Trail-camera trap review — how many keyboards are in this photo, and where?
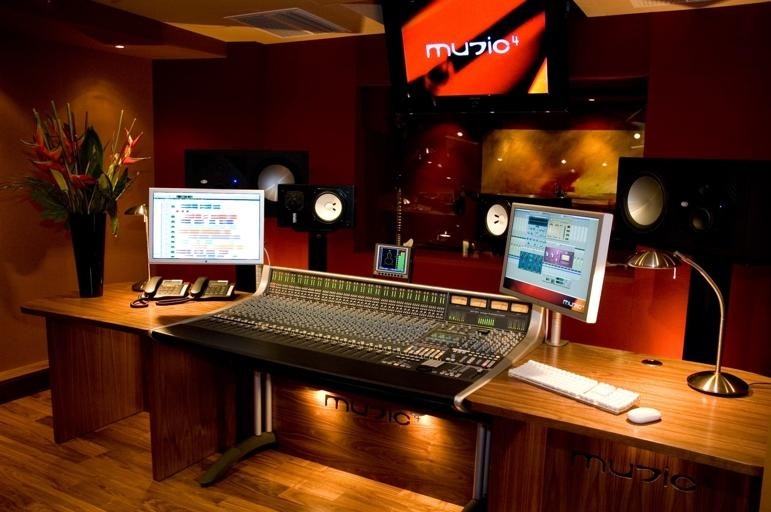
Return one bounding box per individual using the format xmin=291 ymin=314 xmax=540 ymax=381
xmin=508 ymin=360 xmax=641 ymax=415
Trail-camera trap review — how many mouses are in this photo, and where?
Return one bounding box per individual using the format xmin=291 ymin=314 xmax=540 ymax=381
xmin=628 ymin=407 xmax=660 ymax=424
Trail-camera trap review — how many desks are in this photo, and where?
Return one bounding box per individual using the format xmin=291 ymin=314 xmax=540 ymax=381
xmin=18 ymin=279 xmax=769 ymax=511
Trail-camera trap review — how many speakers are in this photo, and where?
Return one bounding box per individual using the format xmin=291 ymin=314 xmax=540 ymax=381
xmin=277 ymin=184 xmax=355 ymax=230
xmin=614 ymin=157 xmax=771 ymax=265
xmin=184 ymin=148 xmax=308 ymax=216
xmin=474 ymin=192 xmax=572 ymax=255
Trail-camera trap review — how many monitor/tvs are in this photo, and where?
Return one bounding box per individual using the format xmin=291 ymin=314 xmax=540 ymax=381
xmin=373 ymin=243 xmax=411 ymax=279
xmin=381 ymin=0 xmax=570 ymax=117
xmin=500 ymin=202 xmax=613 ymax=344
xmin=149 ymin=187 xmax=264 ymax=265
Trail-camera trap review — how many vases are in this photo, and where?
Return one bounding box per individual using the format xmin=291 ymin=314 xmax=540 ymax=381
xmin=67 ymin=212 xmax=107 ymax=297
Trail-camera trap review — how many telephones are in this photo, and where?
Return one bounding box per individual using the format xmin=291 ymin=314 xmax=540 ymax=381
xmin=188 ymin=276 xmax=237 ymax=300
xmin=140 ymin=275 xmax=190 ymax=299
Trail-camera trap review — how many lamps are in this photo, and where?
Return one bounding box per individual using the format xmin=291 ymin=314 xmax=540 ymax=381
xmin=627 ymin=247 xmax=749 ymax=397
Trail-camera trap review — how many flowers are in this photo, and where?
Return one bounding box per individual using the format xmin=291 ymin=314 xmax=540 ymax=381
xmin=0 ymin=99 xmax=152 ymax=241
